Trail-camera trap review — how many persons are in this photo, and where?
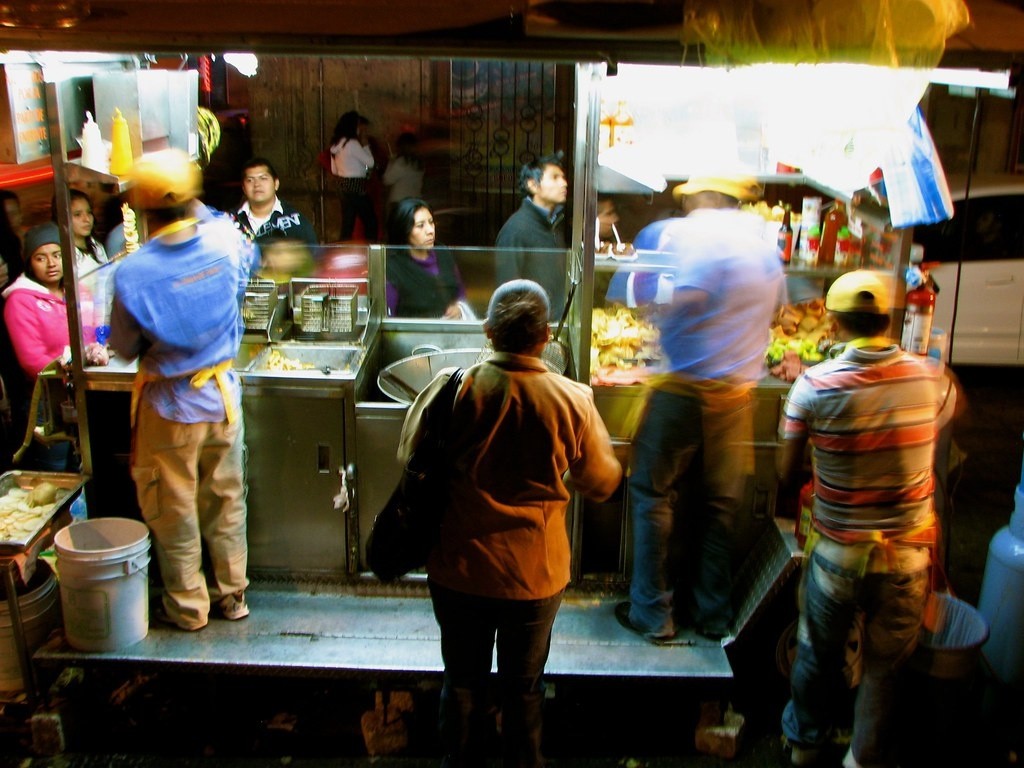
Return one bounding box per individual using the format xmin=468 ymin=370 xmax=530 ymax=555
xmin=109 ymin=148 xmax=260 ymax=634
xmin=396 ymin=279 xmax=622 ymax=768
xmin=0 ymin=187 xmax=221 ymax=471
xmin=603 ymin=171 xmax=787 ymax=642
xmin=778 ymin=271 xmax=956 ymax=768
xmin=231 ymin=155 xmax=328 ymax=295
xmin=383 ymin=197 xmax=465 ymax=320
xmin=495 ymin=151 xmax=619 ymax=322
xmin=329 ymin=110 xmax=424 ymax=247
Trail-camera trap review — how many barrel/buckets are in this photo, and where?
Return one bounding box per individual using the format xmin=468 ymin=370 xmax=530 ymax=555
xmin=0 ymin=559 xmax=57 ymax=705
xmin=54 ymin=518 xmax=151 ymax=651
xmin=795 ymin=480 xmax=815 ymax=549
xmin=903 ymin=593 xmax=989 ymax=756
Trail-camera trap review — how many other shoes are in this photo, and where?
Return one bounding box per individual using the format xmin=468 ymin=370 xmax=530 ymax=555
xmin=218 ymin=593 xmax=249 ymax=619
xmin=677 ymin=608 xmax=724 ymax=640
xmin=151 ymin=594 xmax=208 ymax=630
xmin=615 ymin=601 xmax=676 ymax=637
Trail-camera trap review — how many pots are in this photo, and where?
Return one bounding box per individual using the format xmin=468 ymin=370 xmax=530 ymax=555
xmin=377 ymin=344 xmax=561 ymax=406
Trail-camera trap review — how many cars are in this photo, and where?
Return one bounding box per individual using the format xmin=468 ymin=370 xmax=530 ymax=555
xmin=213 ymin=107 xmax=287 ymax=154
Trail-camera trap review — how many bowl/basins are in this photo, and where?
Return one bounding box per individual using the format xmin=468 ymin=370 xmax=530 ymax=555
xmin=769 ymin=223 xmax=801 ymax=255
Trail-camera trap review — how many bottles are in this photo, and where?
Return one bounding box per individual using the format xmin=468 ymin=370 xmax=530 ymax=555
xmin=81 ymin=112 xmax=109 ymax=176
xmin=834 ymin=226 xmax=851 ymax=265
xmin=804 ymin=226 xmax=819 ymax=266
xmin=776 ymin=205 xmax=793 ymax=263
xmin=818 ymin=200 xmax=848 ymax=264
xmin=909 ymin=245 xmax=924 ymax=270
xmin=929 ymin=328 xmax=948 ymax=370
xmin=850 ymin=220 xmax=898 ymax=269
xmin=111 ymin=108 xmax=134 ymax=175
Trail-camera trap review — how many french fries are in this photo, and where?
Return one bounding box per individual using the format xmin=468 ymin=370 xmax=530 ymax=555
xmin=269 ymin=350 xmax=315 ymax=371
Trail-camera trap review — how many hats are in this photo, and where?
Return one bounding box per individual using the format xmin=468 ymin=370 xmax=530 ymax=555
xmin=674 ymin=177 xmax=758 ymax=200
xmin=826 ymin=270 xmax=891 ymax=313
xmin=25 ymin=222 xmax=60 ymax=259
xmin=130 ymin=150 xmax=201 ymax=208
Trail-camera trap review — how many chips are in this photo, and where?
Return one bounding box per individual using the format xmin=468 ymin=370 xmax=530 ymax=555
xmin=0 ymin=488 xmax=56 ymax=542
xmin=771 ymin=297 xmax=831 ymax=344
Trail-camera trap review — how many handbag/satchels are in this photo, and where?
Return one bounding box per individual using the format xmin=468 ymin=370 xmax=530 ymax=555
xmin=366 ymin=368 xmax=465 ymax=585
xmin=441 ymin=301 xmax=477 ymax=322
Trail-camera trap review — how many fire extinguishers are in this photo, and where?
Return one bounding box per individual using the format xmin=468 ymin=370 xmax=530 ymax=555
xmin=902 ymin=263 xmax=941 ymax=358
